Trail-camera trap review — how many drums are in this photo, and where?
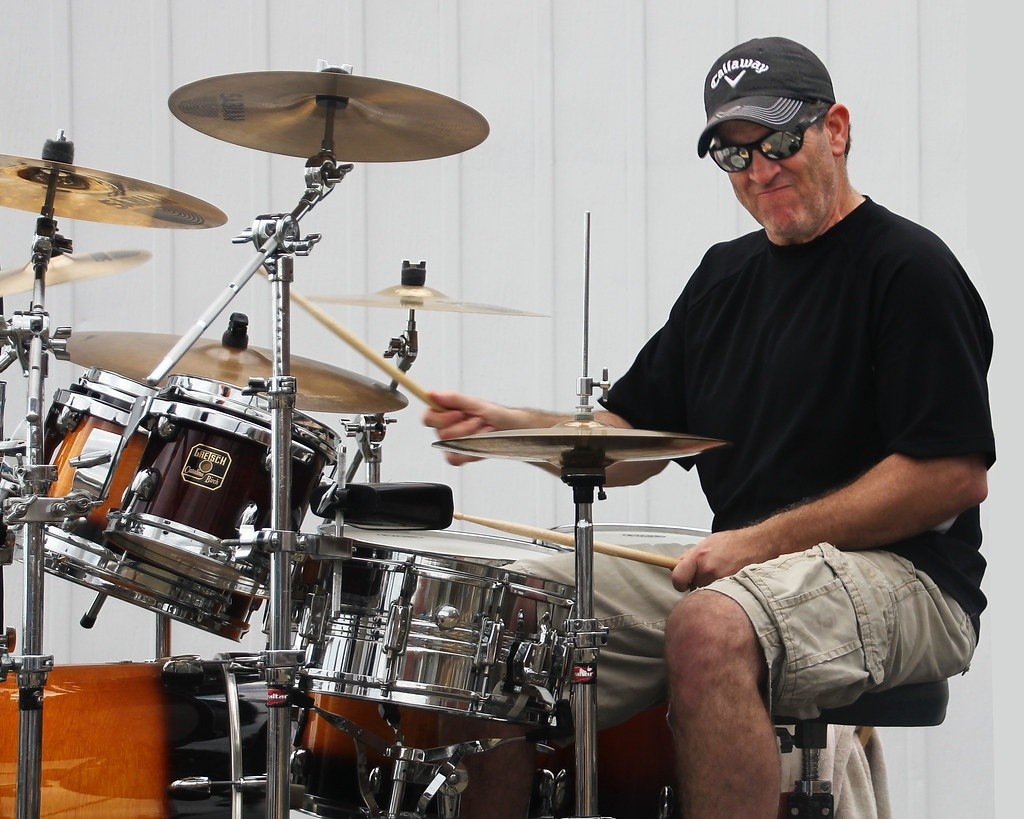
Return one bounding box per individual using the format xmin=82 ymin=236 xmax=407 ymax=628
xmin=528 ymin=523 xmax=720 ymax=818
xmin=274 ymin=532 xmax=579 ymax=731
xmin=287 ymin=691 xmax=556 ymax=819
xmin=108 ymin=371 xmax=347 ymax=601
xmin=9 ymin=366 xmax=260 ymax=645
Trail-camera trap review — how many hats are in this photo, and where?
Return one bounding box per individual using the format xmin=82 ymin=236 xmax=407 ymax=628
xmin=698 ymin=37 xmax=836 ymax=159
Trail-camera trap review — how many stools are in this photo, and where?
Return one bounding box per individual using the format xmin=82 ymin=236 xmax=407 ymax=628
xmin=771 ymin=678 xmax=949 ymax=819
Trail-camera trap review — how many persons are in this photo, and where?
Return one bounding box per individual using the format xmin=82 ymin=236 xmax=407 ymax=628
xmin=424 ymin=38 xmax=997 ymax=819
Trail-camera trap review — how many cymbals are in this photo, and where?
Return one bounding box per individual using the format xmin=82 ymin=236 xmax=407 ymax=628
xmin=433 ymin=416 xmax=729 ymax=461
xmin=288 ymin=283 xmax=551 ymax=318
xmin=0 ymin=250 xmax=153 ymax=293
xmin=55 ymin=329 xmax=407 ymax=416
xmin=0 ymin=153 xmax=230 ymax=230
xmin=166 ymin=69 xmax=491 ymax=163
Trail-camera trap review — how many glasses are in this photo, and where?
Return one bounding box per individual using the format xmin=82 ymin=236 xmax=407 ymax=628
xmin=709 ymin=107 xmax=828 ymax=173
